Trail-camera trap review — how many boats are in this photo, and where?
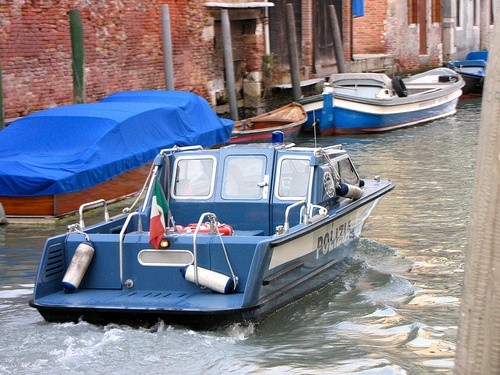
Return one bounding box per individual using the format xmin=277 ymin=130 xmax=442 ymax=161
xmin=29 ymin=131 xmax=397 ymax=326
xmin=0 ymin=90 xmax=307 ymax=224
xmin=446 ymin=51 xmax=487 ymax=95
xmin=297 ymin=67 xmax=466 ymax=134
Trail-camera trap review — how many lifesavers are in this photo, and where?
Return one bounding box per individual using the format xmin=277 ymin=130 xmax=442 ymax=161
xmin=170 ymin=223 xmax=235 ymax=236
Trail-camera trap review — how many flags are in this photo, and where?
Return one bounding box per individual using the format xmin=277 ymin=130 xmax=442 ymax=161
xmin=149 ymin=174 xmax=170 ymax=250
